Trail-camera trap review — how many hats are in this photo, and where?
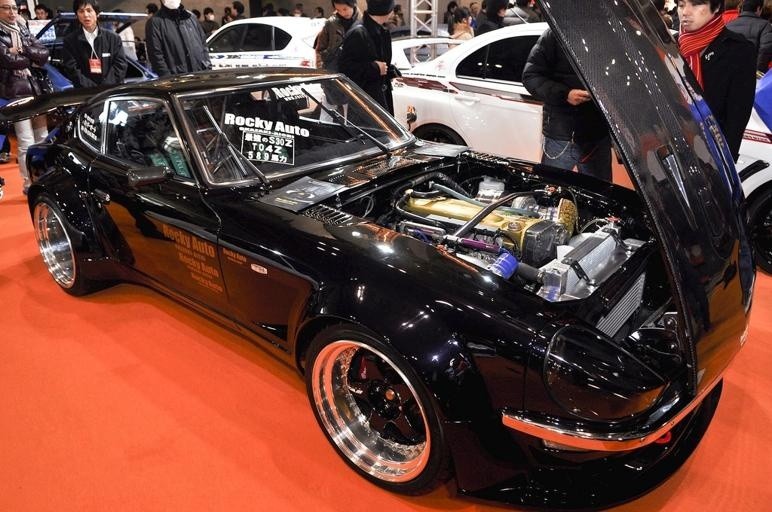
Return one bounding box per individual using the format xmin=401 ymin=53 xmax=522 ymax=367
xmin=365 ymin=1 xmax=395 ymax=16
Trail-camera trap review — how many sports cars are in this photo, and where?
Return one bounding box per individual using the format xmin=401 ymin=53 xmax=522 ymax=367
xmin=5 ymin=2 xmax=760 ymax=508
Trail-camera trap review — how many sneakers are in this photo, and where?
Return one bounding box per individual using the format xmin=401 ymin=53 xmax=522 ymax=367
xmin=1 ymin=151 xmax=11 ymax=163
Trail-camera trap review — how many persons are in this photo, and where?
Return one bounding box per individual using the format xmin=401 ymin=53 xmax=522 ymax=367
xmin=521 ymin=25 xmax=617 ymax=185
xmin=313 ymin=0 xmax=358 ymax=110
xmin=0 ymin=0 xmax=50 ymax=196
xmin=341 ymin=0 xmax=398 ymax=118
xmin=60 ymin=0 xmax=129 ymax=89
xmin=670 ymin=1 xmax=759 ymax=164
xmin=727 ymin=1 xmax=772 ymax=77
xmin=144 ymin=0 xmax=212 ymax=78
xmin=18 ymin=3 xmax=159 ymax=71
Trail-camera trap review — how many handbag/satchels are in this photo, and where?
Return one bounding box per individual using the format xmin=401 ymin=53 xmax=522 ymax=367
xmin=36 ymin=69 xmax=66 ymax=125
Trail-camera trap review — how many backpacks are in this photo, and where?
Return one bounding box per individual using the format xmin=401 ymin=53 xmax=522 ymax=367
xmin=320 ymin=41 xmax=350 ymax=106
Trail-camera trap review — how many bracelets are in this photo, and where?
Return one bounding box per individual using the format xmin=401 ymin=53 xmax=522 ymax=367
xmin=17 ymin=47 xmax=22 ymax=54
